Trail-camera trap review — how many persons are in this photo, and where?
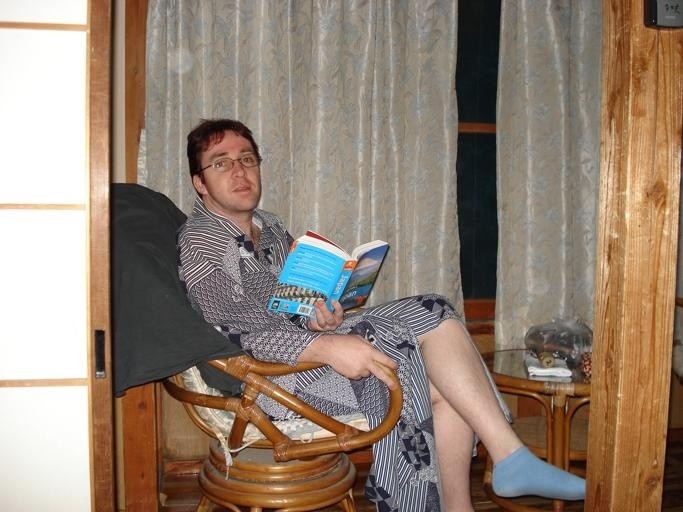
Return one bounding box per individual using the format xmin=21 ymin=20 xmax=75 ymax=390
xmin=174 ymin=118 xmax=590 ymax=511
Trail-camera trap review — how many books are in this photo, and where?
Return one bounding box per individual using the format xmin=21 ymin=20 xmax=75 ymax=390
xmin=265 ymin=228 xmax=389 ymax=321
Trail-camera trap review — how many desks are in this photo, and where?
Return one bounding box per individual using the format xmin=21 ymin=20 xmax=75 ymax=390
xmin=482 ymin=345 xmax=591 ymax=510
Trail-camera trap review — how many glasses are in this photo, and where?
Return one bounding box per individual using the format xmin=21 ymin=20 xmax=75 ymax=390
xmin=198 ymin=152 xmax=260 ymax=172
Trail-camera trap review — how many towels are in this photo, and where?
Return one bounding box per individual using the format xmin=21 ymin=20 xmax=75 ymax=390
xmin=525 ymin=356 xmax=572 ymax=379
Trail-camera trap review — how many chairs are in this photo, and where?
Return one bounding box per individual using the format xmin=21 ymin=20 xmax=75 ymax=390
xmin=109 ymin=182 xmax=402 ymax=511
